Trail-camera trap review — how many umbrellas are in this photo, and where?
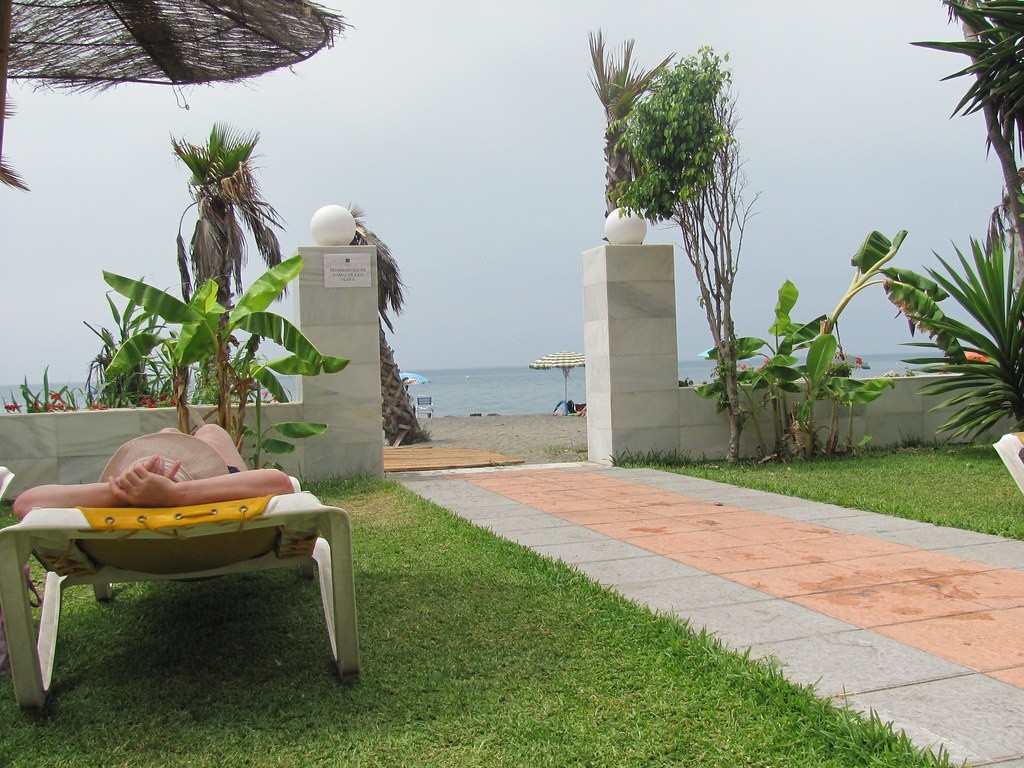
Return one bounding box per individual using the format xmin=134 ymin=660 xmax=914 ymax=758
xmin=831 ymin=351 xmax=870 ymax=370
xmin=529 ymin=350 xmax=586 ymax=415
xmin=698 ymin=347 xmax=715 ymax=357
xmin=399 ymin=371 xmax=432 ymax=394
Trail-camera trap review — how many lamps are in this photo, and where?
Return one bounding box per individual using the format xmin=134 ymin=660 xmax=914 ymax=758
xmin=604 ymin=207 xmax=647 ymax=244
xmin=311 ymin=205 xmax=356 ymax=247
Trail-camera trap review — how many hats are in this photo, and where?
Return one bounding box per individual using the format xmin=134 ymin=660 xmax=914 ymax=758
xmin=99 ymin=432 xmax=230 ymax=484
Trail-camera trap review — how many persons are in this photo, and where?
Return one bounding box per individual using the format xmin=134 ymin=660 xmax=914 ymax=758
xmin=13 ymin=423 xmax=295 ymax=520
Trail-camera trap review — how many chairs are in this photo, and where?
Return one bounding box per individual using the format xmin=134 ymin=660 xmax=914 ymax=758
xmin=0 ymin=476 xmax=361 ymax=708
xmin=416 ymin=397 xmax=433 ymax=419
